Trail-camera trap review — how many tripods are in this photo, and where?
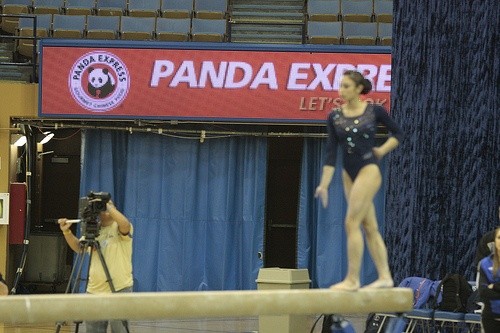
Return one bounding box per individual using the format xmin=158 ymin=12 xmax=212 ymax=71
xmin=54 ymin=218 xmax=132 ymax=333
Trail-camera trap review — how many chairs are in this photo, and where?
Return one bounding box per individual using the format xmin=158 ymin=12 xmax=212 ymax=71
xmin=302 ymin=0 xmax=393 ymax=47
xmin=0 ymin=0 xmax=230 ymax=65
xmin=374 ymin=276 xmax=485 ymax=333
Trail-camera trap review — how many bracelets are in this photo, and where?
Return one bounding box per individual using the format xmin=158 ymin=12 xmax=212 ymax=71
xmin=63 ymin=229 xmax=71 ymax=235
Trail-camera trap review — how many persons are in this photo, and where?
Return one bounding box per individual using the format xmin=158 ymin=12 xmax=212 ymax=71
xmin=315 ymin=70 xmax=404 ymax=292
xmin=476 ymin=227 xmax=500 ymax=333
xmin=58 ymin=200 xmax=134 ymax=333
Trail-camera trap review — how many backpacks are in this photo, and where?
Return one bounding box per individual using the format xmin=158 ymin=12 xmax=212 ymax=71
xmin=430 ymin=273 xmax=472 ymax=327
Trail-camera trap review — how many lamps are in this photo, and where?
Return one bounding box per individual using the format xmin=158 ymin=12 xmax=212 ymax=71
xmin=10 ymin=126 xmax=55 ymax=146
